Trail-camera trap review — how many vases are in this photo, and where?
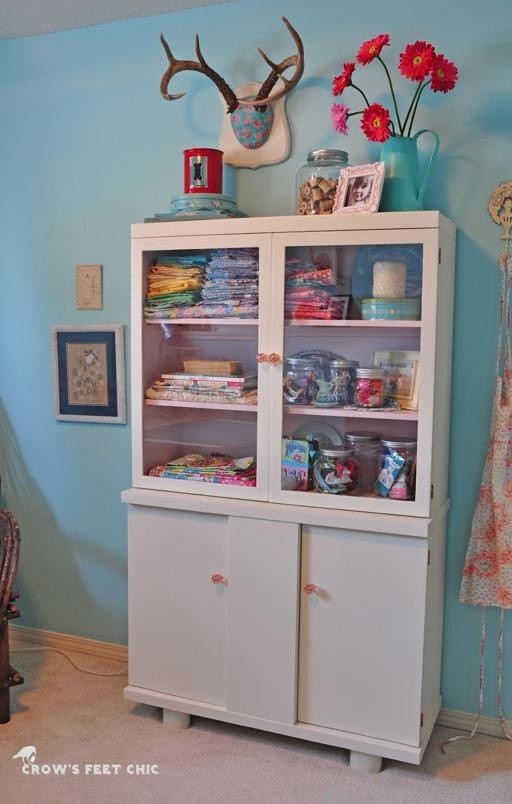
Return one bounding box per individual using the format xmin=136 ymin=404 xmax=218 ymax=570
xmin=363 ymin=132 xmax=445 ymax=210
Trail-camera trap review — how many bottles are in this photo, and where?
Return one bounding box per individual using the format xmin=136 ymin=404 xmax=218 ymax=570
xmin=345 ymin=431 xmax=380 ymax=491
xmin=379 ymin=438 xmax=416 ymax=500
xmin=328 ymin=360 xmax=358 ymax=404
xmin=354 ymin=368 xmax=385 ymax=408
xmin=283 ymin=356 xmax=324 ymax=405
xmin=312 ymin=447 xmax=359 ymax=494
xmin=294 ymin=150 xmax=357 ymax=214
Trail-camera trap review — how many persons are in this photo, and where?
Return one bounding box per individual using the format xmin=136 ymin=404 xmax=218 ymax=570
xmin=349 ymin=175 xmax=373 ymax=205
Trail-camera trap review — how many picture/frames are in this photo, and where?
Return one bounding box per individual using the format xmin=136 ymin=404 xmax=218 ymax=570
xmin=329 ymin=160 xmax=385 ymax=213
xmin=50 ymin=322 xmax=127 ymax=427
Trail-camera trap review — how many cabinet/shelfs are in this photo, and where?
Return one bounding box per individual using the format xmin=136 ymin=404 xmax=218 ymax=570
xmin=124 ymin=206 xmax=452 ymax=774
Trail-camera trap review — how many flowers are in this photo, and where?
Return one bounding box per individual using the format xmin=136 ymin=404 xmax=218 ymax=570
xmin=328 ymin=39 xmax=463 ymax=134
